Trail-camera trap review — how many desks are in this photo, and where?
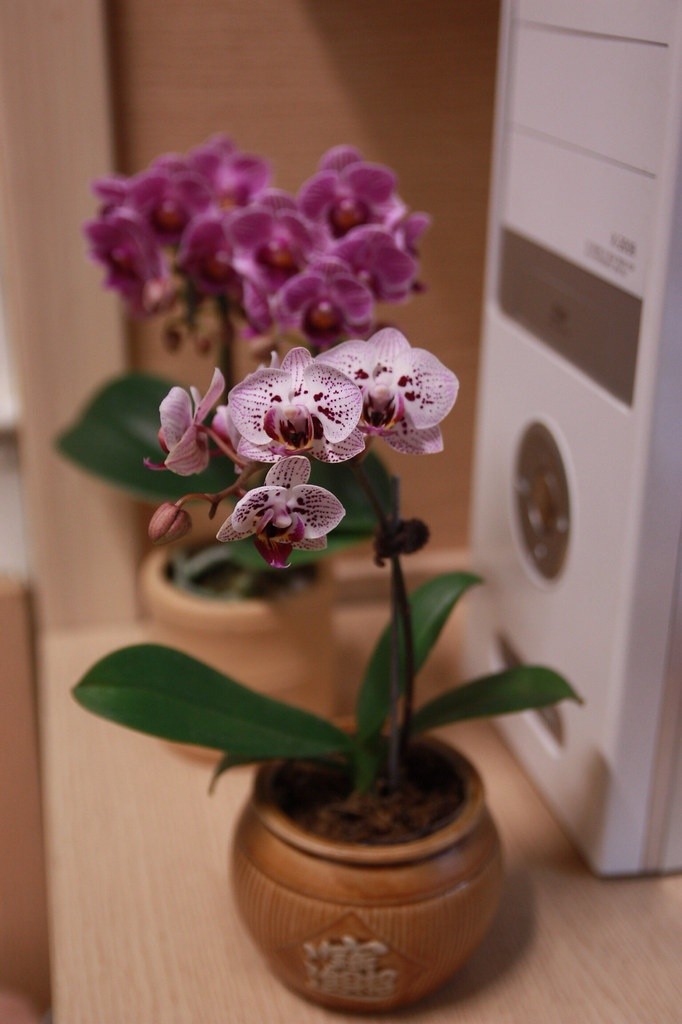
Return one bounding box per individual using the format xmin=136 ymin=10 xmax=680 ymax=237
xmin=36 ymin=553 xmax=682 ymax=1024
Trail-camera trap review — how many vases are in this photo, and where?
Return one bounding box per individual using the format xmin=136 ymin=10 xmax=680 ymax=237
xmin=137 ymin=526 xmax=344 ymax=775
xmin=226 ymin=716 xmax=508 ymax=1014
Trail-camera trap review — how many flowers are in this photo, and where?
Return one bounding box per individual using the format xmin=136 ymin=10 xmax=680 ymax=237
xmin=129 ymin=316 xmax=457 ymax=576
xmin=63 ymin=130 xmax=437 ymax=344
